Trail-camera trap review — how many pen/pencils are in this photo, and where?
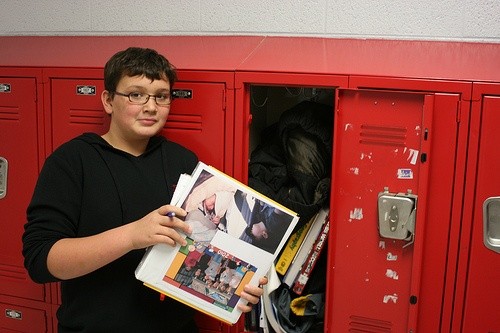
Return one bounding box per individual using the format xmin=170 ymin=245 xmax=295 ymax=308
xmin=167 ymin=212 xmax=176 ymax=217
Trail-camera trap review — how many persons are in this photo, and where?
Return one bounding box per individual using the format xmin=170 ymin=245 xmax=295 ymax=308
xmin=22 ymin=46 xmax=268 ymax=333
xmin=178 ymin=169 xmax=293 ymax=294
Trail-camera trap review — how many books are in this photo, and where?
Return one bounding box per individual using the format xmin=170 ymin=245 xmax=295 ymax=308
xmin=248 ymin=206 xmax=330 ymax=333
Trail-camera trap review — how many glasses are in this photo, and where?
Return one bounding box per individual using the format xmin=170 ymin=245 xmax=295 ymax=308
xmin=113 ymin=91 xmax=173 ymax=105
